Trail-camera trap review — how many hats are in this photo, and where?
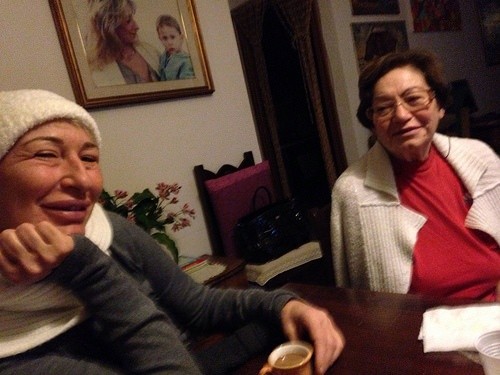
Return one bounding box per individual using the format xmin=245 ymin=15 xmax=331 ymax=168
xmin=0 ymin=89 xmax=103 ymax=159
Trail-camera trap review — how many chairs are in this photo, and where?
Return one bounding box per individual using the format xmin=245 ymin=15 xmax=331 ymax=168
xmin=193 ymin=152 xmax=328 ymax=292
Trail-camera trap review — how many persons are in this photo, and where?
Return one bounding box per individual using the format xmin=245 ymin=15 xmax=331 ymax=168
xmin=155 ymin=16 xmax=195 ymax=80
xmin=331 ymin=48 xmax=500 ymax=304
xmin=84 ymin=0 xmax=162 ymax=87
xmin=0 ymin=88 xmax=345 ymax=375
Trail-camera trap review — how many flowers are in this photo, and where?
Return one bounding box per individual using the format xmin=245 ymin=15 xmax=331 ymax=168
xmin=97 ymin=182 xmax=197 ymax=269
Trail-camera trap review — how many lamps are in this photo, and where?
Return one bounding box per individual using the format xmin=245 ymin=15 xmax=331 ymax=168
xmin=446 ymin=80 xmax=479 ymax=135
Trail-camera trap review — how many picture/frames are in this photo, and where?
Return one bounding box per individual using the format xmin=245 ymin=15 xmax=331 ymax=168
xmin=49 ymin=0 xmax=216 ymax=110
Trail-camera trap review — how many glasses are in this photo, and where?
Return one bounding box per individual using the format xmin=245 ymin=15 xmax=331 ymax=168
xmin=366 ymin=88 xmax=435 ymax=120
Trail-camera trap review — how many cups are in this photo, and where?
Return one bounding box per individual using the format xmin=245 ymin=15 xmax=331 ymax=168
xmin=475 ymin=329 xmax=500 ymax=375
xmin=259 ymin=341 xmax=314 ymax=375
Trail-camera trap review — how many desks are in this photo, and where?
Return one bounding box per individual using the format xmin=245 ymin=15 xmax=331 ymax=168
xmin=181 ymin=255 xmax=246 ymax=290
xmin=187 ymin=283 xmax=500 ymax=375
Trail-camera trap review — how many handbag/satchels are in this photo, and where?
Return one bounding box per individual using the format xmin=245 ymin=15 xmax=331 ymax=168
xmin=232 ymin=196 xmax=314 ymax=265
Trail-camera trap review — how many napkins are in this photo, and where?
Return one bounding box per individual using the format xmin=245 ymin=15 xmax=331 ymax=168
xmin=417 ymin=304 xmax=500 ymax=353
